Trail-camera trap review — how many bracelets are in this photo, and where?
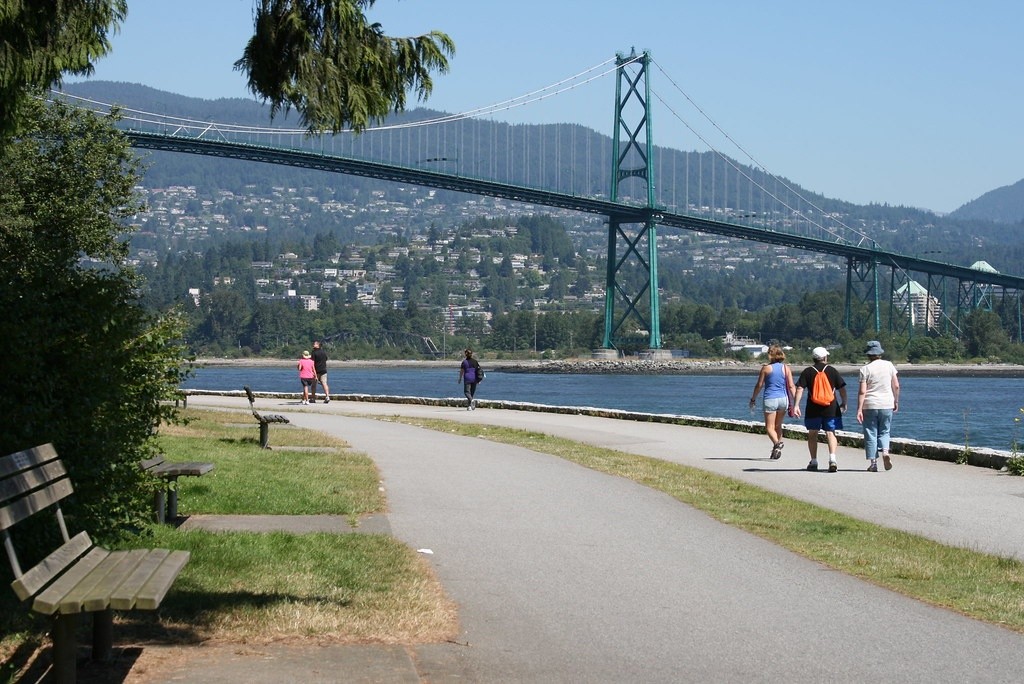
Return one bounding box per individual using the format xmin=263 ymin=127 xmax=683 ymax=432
xmin=894 ymin=402 xmax=899 ymax=403
xmin=751 ymin=399 xmax=755 ymax=402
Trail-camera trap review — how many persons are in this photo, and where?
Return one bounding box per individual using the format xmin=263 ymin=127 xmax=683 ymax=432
xmin=298 ymin=341 xmax=330 ymax=405
xmin=458 ymin=349 xmax=480 ymax=411
xmin=857 ymin=341 xmax=899 ymax=472
xmin=750 ymin=345 xmax=797 ymax=459
xmin=794 ymin=347 xmax=848 ymax=472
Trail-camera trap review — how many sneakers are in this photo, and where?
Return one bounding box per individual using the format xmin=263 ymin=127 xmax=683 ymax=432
xmin=807 ymin=462 xmax=818 ymax=470
xmin=324 ymin=397 xmax=330 ymax=403
xmin=302 ymin=400 xmax=309 ymax=405
xmin=883 ymin=455 xmax=892 ymax=470
xmin=867 ymin=464 xmax=877 ymax=471
xmin=467 ymin=400 xmax=477 ymax=411
xmin=309 ymin=398 xmax=315 ymax=403
xmin=770 ymin=442 xmax=784 ymax=459
xmin=828 ymin=460 xmax=837 ymax=472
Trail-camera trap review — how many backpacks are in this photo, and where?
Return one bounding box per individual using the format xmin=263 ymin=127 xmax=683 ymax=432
xmin=810 ymin=365 xmax=835 ymax=406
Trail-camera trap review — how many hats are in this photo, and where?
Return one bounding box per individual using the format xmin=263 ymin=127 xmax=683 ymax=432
xmin=302 ymin=351 xmax=311 ymax=359
xmin=864 ymin=341 xmax=884 ymax=355
xmin=812 ymin=346 xmax=831 ymax=358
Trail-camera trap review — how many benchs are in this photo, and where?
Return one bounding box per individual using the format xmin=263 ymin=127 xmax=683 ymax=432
xmin=0 ymin=443 xmax=190 ymax=684
xmin=243 ymin=385 xmax=289 ymax=448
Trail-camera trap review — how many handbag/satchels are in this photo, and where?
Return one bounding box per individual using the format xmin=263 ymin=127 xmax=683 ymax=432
xmin=475 ymin=368 xmax=484 ymax=383
xmin=788 ymin=406 xmax=794 ymax=418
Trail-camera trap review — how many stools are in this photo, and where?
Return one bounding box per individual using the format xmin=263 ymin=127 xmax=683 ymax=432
xmin=140 ymin=456 xmax=214 ymax=523
